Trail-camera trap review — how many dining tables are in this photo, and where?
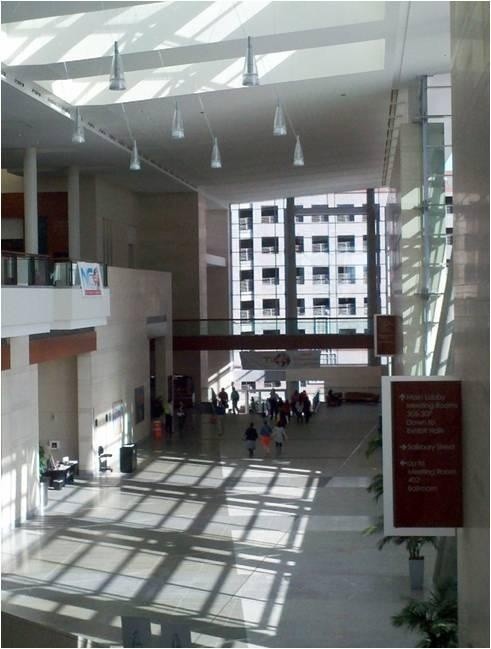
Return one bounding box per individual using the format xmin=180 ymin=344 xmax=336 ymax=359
xmin=361 ymin=513 xmax=438 ymax=591
xmin=39 ymin=443 xmax=49 ymax=507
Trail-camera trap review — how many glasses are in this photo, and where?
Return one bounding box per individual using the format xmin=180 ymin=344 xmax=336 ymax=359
xmin=54 ymin=36 xmax=306 ymax=171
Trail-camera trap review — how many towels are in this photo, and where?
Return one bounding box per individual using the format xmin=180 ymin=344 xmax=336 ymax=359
xmin=39 ymin=482 xmax=48 ymax=508
xmin=120 ymin=443 xmax=138 ymax=473
xmin=53 ymin=479 xmax=63 ymax=490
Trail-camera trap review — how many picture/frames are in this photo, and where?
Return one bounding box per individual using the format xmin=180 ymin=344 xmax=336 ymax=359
xmin=48 ymin=460 xmax=78 ymax=486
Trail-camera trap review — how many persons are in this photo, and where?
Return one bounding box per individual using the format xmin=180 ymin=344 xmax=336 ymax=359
xmin=217 ymin=387 xmax=228 ymax=409
xmin=245 ymin=422 xmax=258 ymax=457
xmin=175 ymin=400 xmax=186 ymax=436
xmin=163 ymin=400 xmax=174 ymax=436
xmin=258 ymin=421 xmax=272 ymax=454
xmin=230 ymin=387 xmax=239 ymax=416
xmin=271 ymin=420 xmax=288 ymax=453
xmin=215 ymin=400 xmax=226 ymax=436
xmin=264 ymin=387 xmax=312 ymax=426
xmin=328 ymin=389 xmax=342 ymax=407
